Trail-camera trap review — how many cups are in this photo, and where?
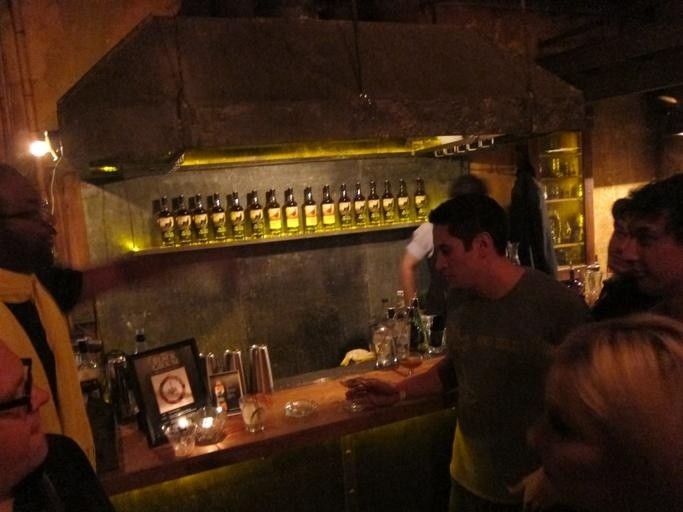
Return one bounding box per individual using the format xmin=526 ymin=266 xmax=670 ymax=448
xmin=221 ymin=349 xmax=247 ymax=399
xmin=239 ymin=394 xmax=271 ymax=433
xmin=193 ymin=407 xmax=226 ymax=444
xmin=198 ymin=351 xmax=218 ymax=402
xmin=247 ymin=343 xmax=273 ymax=394
xmin=160 ymin=421 xmax=196 ymax=458
xmin=373 ymin=330 xmax=396 ymax=367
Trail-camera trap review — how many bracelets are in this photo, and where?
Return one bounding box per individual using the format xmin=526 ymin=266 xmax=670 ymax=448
xmin=397 ymin=380 xmax=407 ymax=401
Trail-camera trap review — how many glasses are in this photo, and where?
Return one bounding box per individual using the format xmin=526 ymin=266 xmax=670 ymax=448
xmin=0 ymin=201 xmax=50 ymax=222
xmin=0 ymin=357 xmax=32 ymax=410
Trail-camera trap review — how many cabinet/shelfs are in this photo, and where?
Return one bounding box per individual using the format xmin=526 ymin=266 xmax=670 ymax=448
xmin=531 ymin=126 xmax=596 ymax=282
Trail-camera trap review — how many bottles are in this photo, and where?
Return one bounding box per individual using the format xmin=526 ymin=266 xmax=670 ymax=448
xmin=536 ymin=156 xmax=586 ymax=266
xmin=147 ymin=177 xmax=436 ymax=250
xmin=75 ymin=339 xmax=109 ymax=413
xmin=586 ymin=267 xmax=602 ymax=304
xmin=131 ymin=333 xmax=151 ymax=354
xmin=377 ymin=289 xmax=445 ymax=360
xmin=565 ymin=270 xmax=584 ymax=302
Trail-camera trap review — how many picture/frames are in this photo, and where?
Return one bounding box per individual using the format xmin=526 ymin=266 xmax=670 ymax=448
xmin=127 ymin=338 xmax=216 ymax=448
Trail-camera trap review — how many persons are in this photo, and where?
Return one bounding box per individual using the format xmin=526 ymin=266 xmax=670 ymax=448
xmin=526 ymin=312 xmax=682 ymax=512
xmin=399 ymin=173 xmax=490 ymax=346
xmin=0 ymin=162 xmax=172 ymax=475
xmin=622 ymin=169 xmax=682 ymax=319
xmin=590 ymin=198 xmax=663 ymax=322
xmin=0 ymin=336 xmax=116 ymax=512
xmin=340 ymin=193 xmax=589 ymax=512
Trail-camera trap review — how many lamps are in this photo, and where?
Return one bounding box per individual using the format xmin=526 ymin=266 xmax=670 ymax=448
xmin=28 ymin=127 xmax=65 ymax=162
